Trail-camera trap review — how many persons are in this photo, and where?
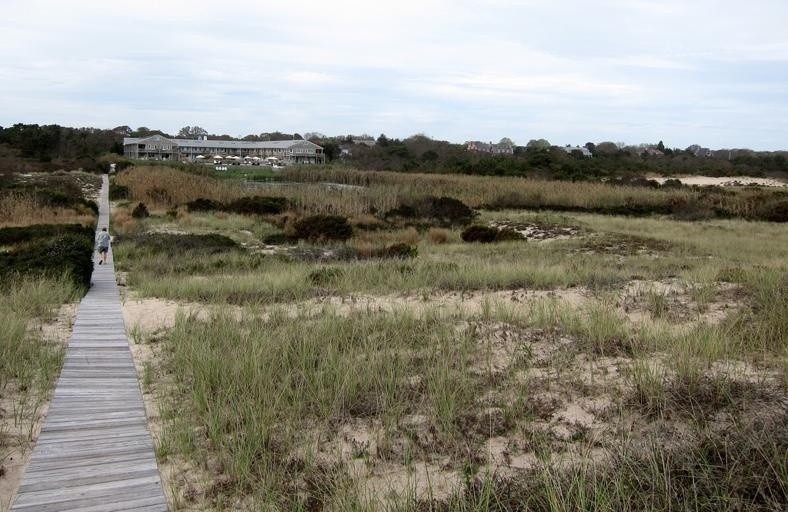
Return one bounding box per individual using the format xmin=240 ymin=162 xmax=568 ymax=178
xmin=97 ymin=226 xmax=111 ymax=266
